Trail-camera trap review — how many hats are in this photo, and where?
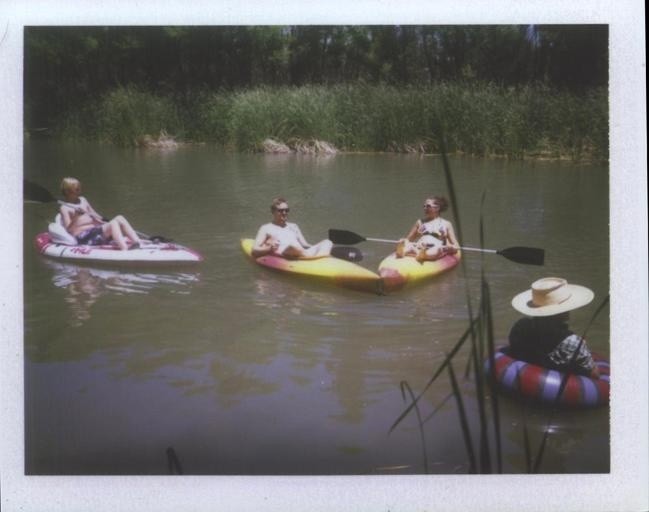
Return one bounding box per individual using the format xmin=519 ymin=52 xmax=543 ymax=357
xmin=511 ymin=277 xmax=595 ymax=317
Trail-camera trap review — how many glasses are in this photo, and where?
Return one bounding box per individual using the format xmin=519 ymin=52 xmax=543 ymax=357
xmin=423 ymin=204 xmax=439 ymax=209
xmin=275 ymin=208 xmax=290 ymax=213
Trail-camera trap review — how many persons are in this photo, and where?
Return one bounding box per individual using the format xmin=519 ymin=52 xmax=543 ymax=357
xmin=60 ymin=176 xmax=143 ymax=250
xmin=396 ymin=196 xmax=458 ymax=261
xmin=507 ymin=277 xmax=599 ymax=379
xmin=252 ymin=199 xmax=333 ymax=259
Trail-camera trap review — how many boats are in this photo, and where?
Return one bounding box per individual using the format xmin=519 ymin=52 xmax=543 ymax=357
xmin=378 ymin=241 xmax=460 ymax=291
xmin=36 ymin=231 xmax=202 ymax=263
xmin=241 ymin=237 xmax=384 ymax=296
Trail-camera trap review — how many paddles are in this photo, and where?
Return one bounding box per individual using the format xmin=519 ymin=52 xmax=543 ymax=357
xmin=329 ymin=229 xmax=544 ymax=266
xmin=57 ymin=199 xmax=173 ymax=242
xmin=304 ymin=246 xmax=362 ymax=261
